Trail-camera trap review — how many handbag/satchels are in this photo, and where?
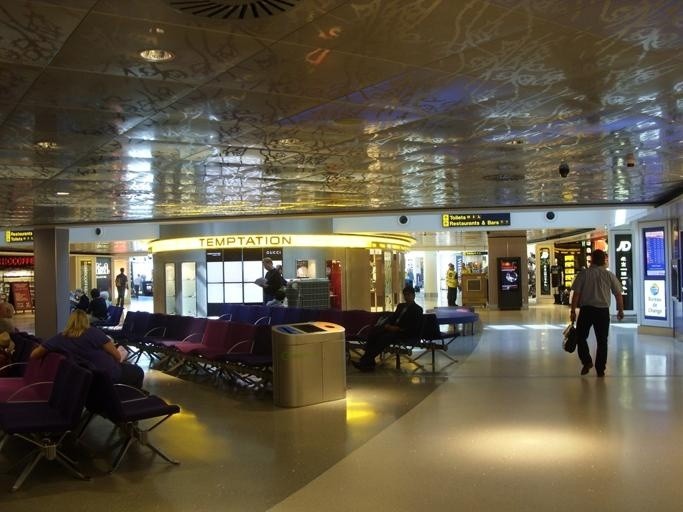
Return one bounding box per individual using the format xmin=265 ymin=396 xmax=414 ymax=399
xmin=364 ymin=325 xmax=386 ymax=339
xmin=0 ymin=344 xmax=16 ymax=375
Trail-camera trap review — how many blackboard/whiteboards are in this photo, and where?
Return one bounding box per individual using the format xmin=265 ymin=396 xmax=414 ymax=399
xmin=11 ymin=282 xmax=32 ymax=310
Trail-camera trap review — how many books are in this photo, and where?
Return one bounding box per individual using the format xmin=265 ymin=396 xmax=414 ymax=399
xmin=68 ymin=291 xmax=77 ymax=303
xmin=252 ymin=277 xmax=266 ymax=288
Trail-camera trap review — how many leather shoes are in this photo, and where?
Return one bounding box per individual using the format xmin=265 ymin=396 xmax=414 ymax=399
xmin=581 ymin=361 xmax=593 ymax=375
xmin=351 ymin=360 xmax=376 ymax=370
xmin=597 ymin=372 xmax=605 ymax=377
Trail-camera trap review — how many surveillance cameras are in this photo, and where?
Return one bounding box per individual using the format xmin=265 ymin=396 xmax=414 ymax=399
xmin=95 ymin=226 xmax=103 ymax=236
xmin=558 ymin=164 xmax=570 ymax=178
xmin=627 ymin=155 xmax=634 ymax=167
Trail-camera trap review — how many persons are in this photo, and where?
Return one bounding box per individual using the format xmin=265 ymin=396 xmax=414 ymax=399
xmin=259 ymin=257 xmax=283 ymax=306
xmin=466 ymin=262 xmax=474 ymax=274
xmin=569 ymin=249 xmax=625 ymax=377
xmin=459 ymin=263 xmax=468 ymax=276
xmin=30 ymin=309 xmax=143 ymax=386
xmin=115 ymin=268 xmax=127 ymax=308
xmin=274 ymin=265 xmax=286 ymax=286
xmin=68 ymin=288 xmax=88 ymax=315
xmin=98 ymin=291 xmax=109 ymax=309
xmin=406 ymin=269 xmax=414 ymax=281
xmin=133 ymin=274 xmax=142 ymax=299
xmin=0 ymin=302 xmax=16 ymax=377
xmin=446 ymin=264 xmax=461 ymax=306
xmin=85 ymin=288 xmax=108 ymax=325
xmin=350 ymin=286 xmax=422 ymax=370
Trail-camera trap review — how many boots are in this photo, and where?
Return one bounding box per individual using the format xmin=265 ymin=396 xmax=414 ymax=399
xmin=121 ymin=304 xmax=125 ymax=309
xmin=116 ymin=304 xmax=119 ymax=307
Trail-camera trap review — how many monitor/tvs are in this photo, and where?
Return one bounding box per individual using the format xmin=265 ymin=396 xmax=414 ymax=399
xmin=289 ymin=323 xmax=326 ymax=334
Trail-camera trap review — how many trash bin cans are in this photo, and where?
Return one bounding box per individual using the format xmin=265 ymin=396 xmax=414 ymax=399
xmin=142 ymin=281 xmax=153 ymax=296
xmin=271 ymin=322 xmax=347 ymax=407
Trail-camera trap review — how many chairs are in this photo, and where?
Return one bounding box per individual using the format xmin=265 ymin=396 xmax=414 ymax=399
xmin=0 ymin=319 xmax=181 ymax=493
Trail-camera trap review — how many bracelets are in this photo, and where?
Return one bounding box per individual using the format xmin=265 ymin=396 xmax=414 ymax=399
xmin=570 ymin=311 xmax=575 ymax=314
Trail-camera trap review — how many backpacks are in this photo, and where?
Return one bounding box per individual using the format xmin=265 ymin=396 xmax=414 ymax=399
xmin=561 ymin=322 xmax=577 ymax=354
